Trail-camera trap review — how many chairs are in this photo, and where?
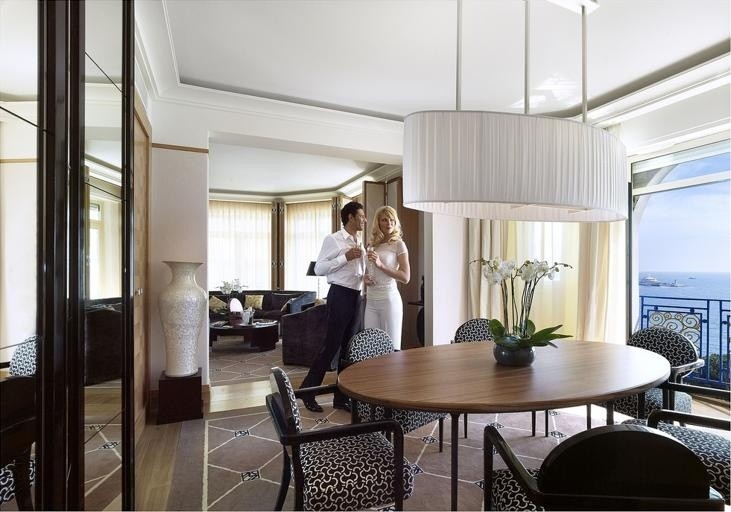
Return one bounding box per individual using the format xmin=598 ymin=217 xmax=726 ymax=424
xmin=2 ymin=373 xmax=35 ymax=510
xmin=265 ymin=367 xmax=414 ymax=512
xmin=451 ymin=319 xmax=536 ymax=437
xmin=340 ymin=328 xmax=448 ymax=452
xmin=623 ymin=409 xmax=729 ymax=502
xmin=477 ymin=426 xmax=722 ymax=511
xmin=587 ymin=328 xmax=704 ymax=428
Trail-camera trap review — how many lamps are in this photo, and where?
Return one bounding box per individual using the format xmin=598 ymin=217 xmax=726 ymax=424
xmin=403 ymin=0 xmax=628 ymax=222
xmin=306 ymin=261 xmax=324 ymax=299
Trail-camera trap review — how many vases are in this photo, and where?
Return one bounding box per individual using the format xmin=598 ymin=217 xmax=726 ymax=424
xmin=157 ymin=261 xmax=207 ymax=378
xmin=493 ymin=346 xmax=534 ymax=367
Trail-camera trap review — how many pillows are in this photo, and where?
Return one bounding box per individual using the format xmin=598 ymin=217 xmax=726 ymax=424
xmin=315 ymin=298 xmax=325 ymax=306
xmin=281 ymin=298 xmax=296 ymax=313
xmin=210 ymin=294 xmax=227 ymax=315
xmin=245 ymin=294 xmax=264 ymax=310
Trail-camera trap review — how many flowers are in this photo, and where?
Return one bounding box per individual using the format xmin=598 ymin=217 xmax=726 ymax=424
xmin=466 ymin=257 xmax=574 ymax=348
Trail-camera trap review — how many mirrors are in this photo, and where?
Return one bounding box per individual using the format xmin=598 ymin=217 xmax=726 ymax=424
xmin=81 ymin=1 xmax=126 ymax=511
xmin=1 ymin=0 xmax=51 ymax=509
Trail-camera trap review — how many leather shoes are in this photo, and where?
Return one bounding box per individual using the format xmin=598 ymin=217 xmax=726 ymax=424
xmin=333 ymin=399 xmax=352 ymax=414
xmin=296 ymin=387 xmax=324 ymax=413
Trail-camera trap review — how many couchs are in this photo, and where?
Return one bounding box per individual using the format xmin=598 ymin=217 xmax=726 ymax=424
xmin=209 ymin=291 xmax=317 ymax=320
xmin=282 ymin=302 xmax=341 ymax=366
xmin=84 ymin=298 xmax=122 ymax=387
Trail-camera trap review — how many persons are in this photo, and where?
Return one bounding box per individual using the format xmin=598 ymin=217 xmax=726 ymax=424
xmin=294 ymin=202 xmax=378 ymax=412
xmin=364 ymin=206 xmax=411 ymax=351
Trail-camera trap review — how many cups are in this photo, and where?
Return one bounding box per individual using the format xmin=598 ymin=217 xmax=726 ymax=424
xmin=352 ymin=241 xmax=362 ymax=277
xmin=366 ymin=245 xmax=374 ymax=281
